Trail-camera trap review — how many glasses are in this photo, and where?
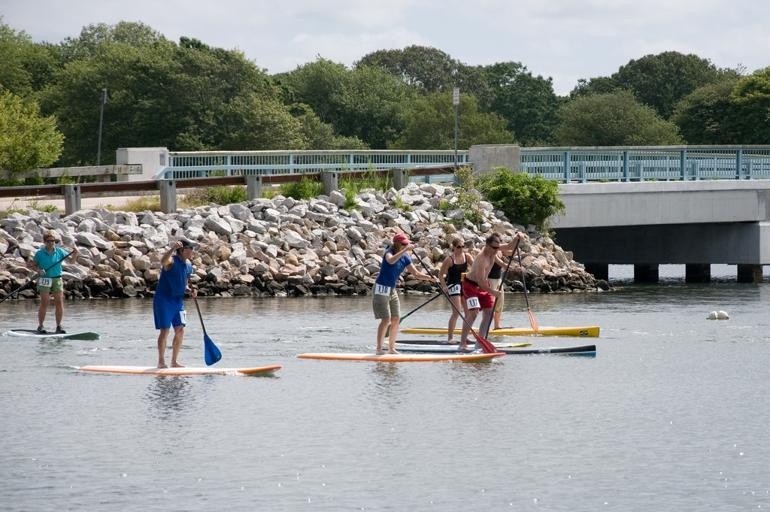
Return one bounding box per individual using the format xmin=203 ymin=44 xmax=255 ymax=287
xmin=490 ymin=245 xmax=499 ymax=249
xmin=456 ymin=245 xmax=464 ymax=248
xmin=46 ymin=239 xmax=55 ymax=242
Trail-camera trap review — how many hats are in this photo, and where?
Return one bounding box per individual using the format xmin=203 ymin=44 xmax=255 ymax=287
xmin=393 ymin=234 xmax=413 ymax=244
xmin=176 ymin=238 xmax=196 ymax=255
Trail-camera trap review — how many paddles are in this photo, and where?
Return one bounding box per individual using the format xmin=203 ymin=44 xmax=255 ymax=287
xmin=517 ymin=245 xmax=538 ymax=331
xmin=178 ymin=247 xmax=221 ymax=365
xmin=413 ymin=248 xmax=499 ymax=352
xmin=385 ymin=280 xmax=460 ymax=338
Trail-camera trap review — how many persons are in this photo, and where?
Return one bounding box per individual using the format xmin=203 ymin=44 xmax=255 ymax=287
xmin=459 ymin=231 xmax=526 ymax=352
xmin=150 ymin=240 xmax=200 ymax=369
xmin=370 ymin=233 xmax=440 ymax=355
xmin=440 ymin=236 xmax=476 ymax=344
xmin=30 ymin=230 xmax=80 ymax=336
xmin=485 ymin=232 xmax=523 ymax=331
